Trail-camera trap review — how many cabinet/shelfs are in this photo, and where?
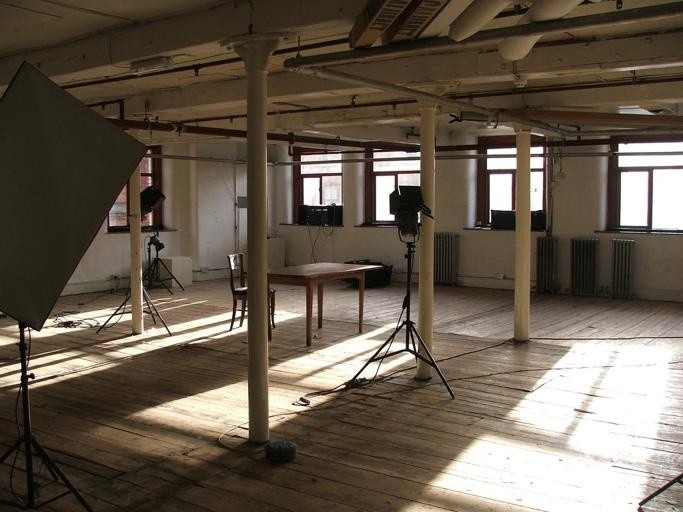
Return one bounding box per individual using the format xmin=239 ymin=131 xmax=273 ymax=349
xmin=154 ymin=255 xmax=193 ymax=288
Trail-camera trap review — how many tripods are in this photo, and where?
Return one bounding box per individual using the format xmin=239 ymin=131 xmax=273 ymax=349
xmin=96 ymin=287 xmax=172 ymax=336
xmin=0 ymin=321 xmax=94 ymax=512
xmin=143 ymin=250 xmax=184 ymax=291
xmin=345 ymin=241 xmax=456 ymax=399
xmin=638 ymin=473 xmax=683 ymax=507
xmin=124 ymin=245 xmax=173 ymax=295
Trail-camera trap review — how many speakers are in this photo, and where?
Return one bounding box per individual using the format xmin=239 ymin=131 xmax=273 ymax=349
xmin=299 ymin=204 xmax=342 ymax=226
xmin=141 ymin=185 xmax=166 ymax=221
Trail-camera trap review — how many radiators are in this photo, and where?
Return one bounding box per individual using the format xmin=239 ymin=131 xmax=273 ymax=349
xmin=434 ymin=230 xmax=460 ymax=288
xmin=536 ymin=235 xmax=636 ymax=302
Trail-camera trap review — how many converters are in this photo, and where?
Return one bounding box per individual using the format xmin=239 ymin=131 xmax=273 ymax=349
xmin=110 ymin=289 xmax=114 ymax=293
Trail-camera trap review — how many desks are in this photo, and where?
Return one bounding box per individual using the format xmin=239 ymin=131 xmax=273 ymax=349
xmin=267 ymin=262 xmax=384 ymax=345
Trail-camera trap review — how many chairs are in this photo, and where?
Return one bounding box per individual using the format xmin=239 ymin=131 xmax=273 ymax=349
xmin=226 ymin=253 xmax=278 ymax=332
